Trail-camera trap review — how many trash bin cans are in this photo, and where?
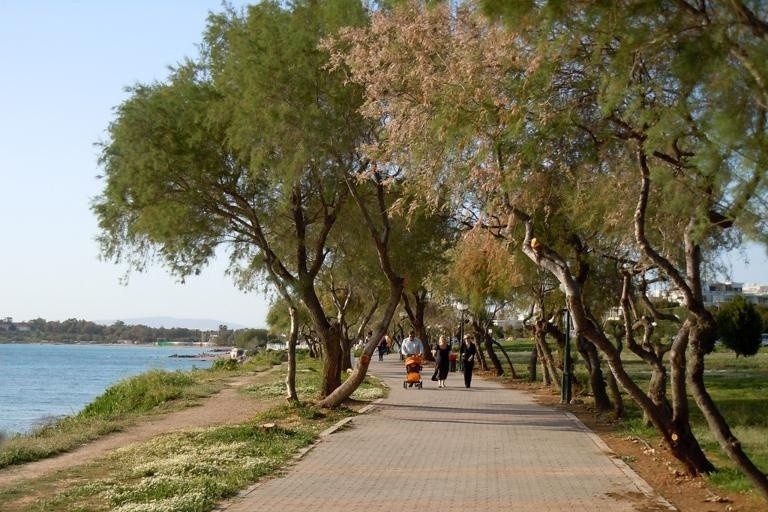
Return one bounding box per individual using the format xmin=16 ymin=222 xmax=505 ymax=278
xmin=448 ymin=353 xmax=456 ymax=372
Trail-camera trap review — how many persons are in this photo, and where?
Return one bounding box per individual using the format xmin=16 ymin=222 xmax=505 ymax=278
xmin=384 ymin=334 xmax=392 ymax=356
xmin=362 ymin=331 xmax=373 ymax=344
xmin=432 ymin=334 xmax=451 ymax=388
xmin=458 ymin=332 xmax=476 ymax=388
xmin=400 ymin=330 xmax=424 ymax=388
xmin=377 ymin=336 xmax=386 ymax=361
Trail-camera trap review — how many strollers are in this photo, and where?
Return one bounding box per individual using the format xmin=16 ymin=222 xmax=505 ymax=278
xmin=402 ymin=354 xmax=423 ymax=390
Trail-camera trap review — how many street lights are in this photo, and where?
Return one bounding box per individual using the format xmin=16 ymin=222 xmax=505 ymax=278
xmin=559 ymin=275 xmax=579 ymax=404
xmin=456 ymin=301 xmax=468 ymax=372
